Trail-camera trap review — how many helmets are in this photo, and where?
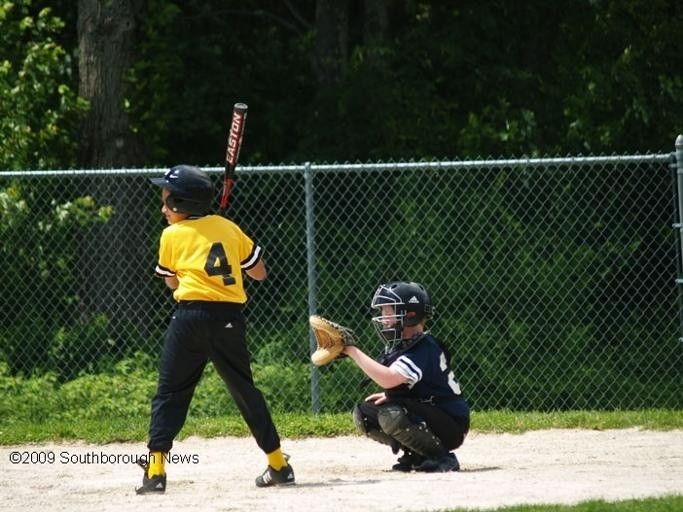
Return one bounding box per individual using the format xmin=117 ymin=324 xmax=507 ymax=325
xmin=148 ymin=165 xmax=215 ymax=217
xmin=371 ymin=281 xmax=433 ymax=357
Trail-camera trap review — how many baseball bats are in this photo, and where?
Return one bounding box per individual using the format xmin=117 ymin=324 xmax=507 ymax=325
xmin=220 ymin=103 xmax=248 ymax=215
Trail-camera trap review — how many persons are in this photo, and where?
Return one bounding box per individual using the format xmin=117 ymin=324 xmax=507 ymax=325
xmin=132 ymin=164 xmax=296 ymax=496
xmin=309 ymin=280 xmax=471 ymax=472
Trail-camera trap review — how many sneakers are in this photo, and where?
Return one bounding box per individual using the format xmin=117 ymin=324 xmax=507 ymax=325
xmin=392 ymin=452 xmax=460 ymax=472
xmin=255 ymin=453 xmax=296 ymax=488
xmin=134 ymin=459 xmax=167 ymax=494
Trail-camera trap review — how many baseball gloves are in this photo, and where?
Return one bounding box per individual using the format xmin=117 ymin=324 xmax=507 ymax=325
xmin=310 ymin=314 xmax=356 ymax=365
